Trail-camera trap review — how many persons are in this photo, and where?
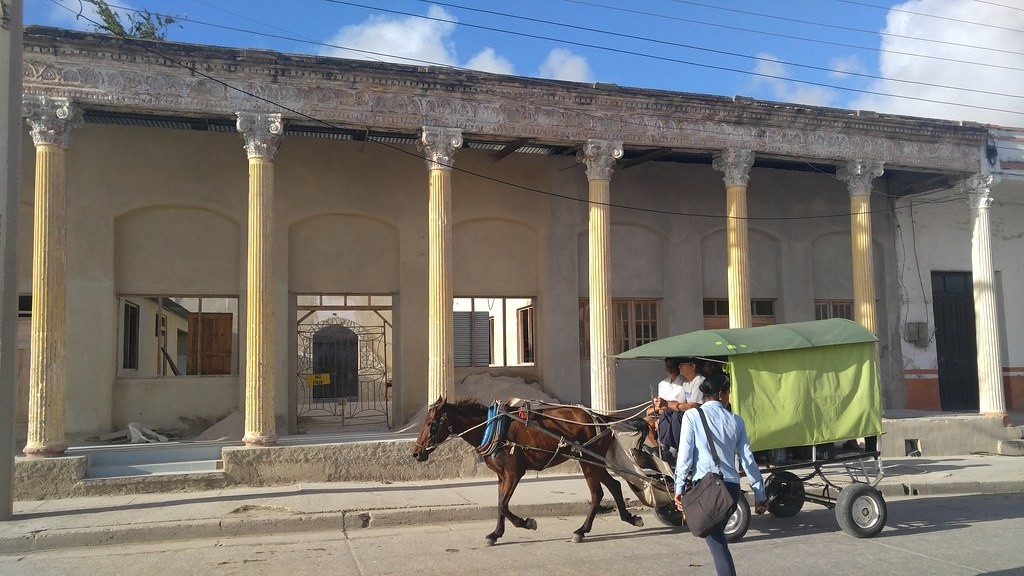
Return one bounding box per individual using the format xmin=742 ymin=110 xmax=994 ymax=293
xmin=673 ymin=372 xmax=770 ymax=576
xmin=646 ymin=357 xmax=727 ymax=469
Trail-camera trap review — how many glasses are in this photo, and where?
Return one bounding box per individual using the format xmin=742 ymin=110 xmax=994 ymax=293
xmin=720 ymin=375 xmax=729 ymax=391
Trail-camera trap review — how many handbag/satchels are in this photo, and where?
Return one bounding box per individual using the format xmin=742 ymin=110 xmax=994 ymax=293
xmin=680 ymin=472 xmax=738 ymax=538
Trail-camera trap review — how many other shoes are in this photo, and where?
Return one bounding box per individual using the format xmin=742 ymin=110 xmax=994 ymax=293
xmin=652 ymin=450 xmax=676 ymax=469
xmin=642 ymin=444 xmax=668 ymax=458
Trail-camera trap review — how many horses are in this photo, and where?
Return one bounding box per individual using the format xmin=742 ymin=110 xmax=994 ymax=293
xmin=410 ymin=396 xmax=651 ymax=546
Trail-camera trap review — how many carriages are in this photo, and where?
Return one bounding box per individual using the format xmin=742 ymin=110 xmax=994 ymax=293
xmin=410 ymin=317 xmax=887 ymax=547
